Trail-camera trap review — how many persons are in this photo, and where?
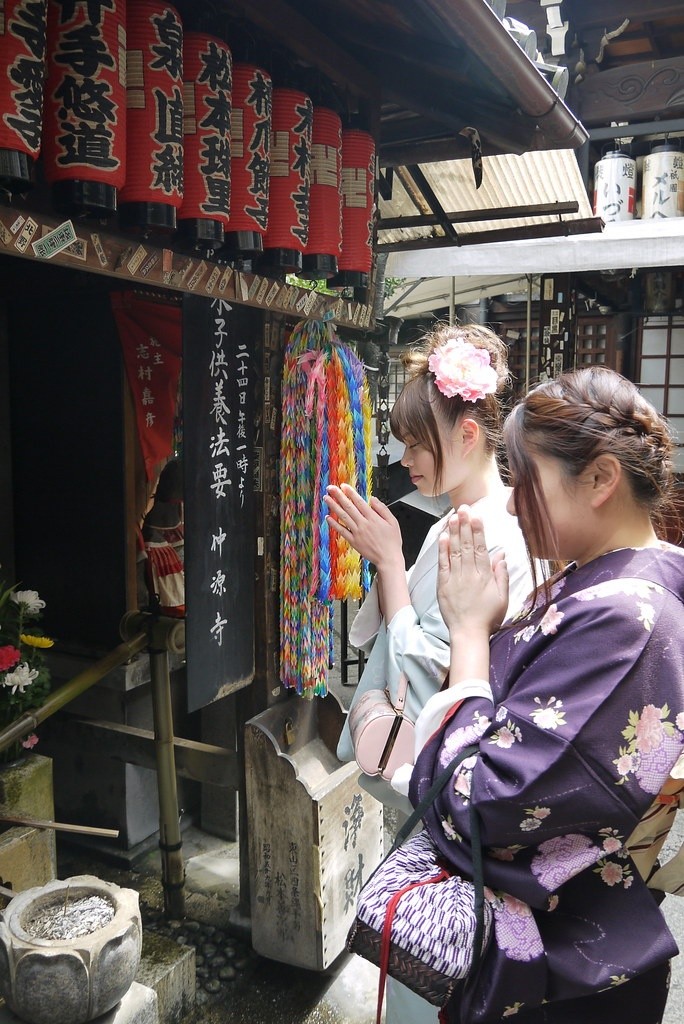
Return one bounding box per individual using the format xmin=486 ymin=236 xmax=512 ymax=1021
xmin=323 ymin=326 xmax=684 ymax=1024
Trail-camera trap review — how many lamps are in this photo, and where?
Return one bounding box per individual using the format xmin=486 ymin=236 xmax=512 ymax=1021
xmin=643 ymin=134 xmax=684 ymax=217
xmin=592 ymin=140 xmax=636 ymax=219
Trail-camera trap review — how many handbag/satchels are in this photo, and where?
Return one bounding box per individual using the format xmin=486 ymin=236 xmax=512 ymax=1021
xmin=346 ymin=742 xmax=496 ymax=1007
xmin=348 ymin=671 xmax=416 ymax=781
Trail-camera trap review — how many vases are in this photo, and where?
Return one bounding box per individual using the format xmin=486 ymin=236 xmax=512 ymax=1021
xmin=0 ymin=755 xmax=59 ymax=880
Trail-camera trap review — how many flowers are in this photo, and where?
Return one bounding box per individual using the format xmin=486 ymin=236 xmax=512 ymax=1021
xmin=0 ymin=580 xmax=52 ymax=757
xmin=429 ymin=339 xmax=497 ymax=402
